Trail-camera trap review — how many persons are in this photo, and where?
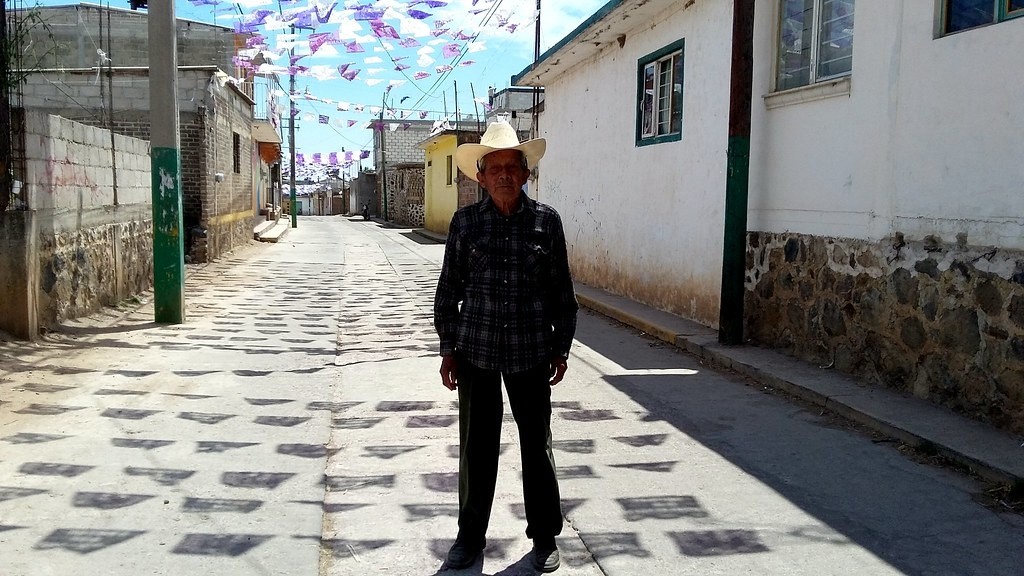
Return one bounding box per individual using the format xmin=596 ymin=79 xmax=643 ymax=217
xmin=432 ymin=122 xmax=578 ymax=572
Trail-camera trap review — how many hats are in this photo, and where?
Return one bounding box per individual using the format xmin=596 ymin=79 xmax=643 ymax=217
xmin=454 ymin=120 xmax=546 ymax=183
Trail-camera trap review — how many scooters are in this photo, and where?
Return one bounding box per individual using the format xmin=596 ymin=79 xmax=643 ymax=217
xmin=360 ymin=199 xmax=371 ymax=221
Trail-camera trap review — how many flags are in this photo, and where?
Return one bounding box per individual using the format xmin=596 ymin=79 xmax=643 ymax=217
xmin=187 ymin=0 xmax=540 ymax=196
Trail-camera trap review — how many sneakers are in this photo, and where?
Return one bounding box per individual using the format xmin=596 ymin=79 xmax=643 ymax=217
xmin=446 ymin=536 xmax=487 ymax=569
xmin=531 ymin=534 xmax=560 ymax=571
